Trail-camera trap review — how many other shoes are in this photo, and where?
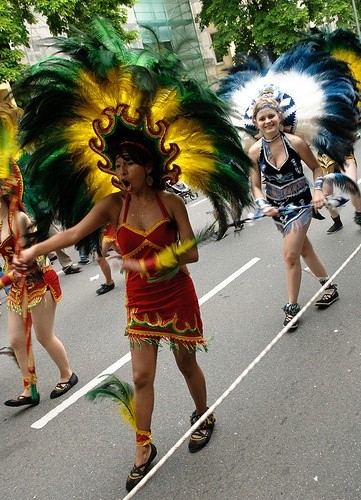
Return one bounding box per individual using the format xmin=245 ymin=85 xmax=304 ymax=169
xmin=217 ymin=226 xmax=230 ymax=240
xmin=78 ymin=258 xmax=90 ymax=265
xmin=4 ymin=393 xmax=40 ymax=407
xmin=50 ymin=372 xmax=78 ymax=399
xmin=315 ymin=284 xmax=340 ymax=306
xmin=355 ymin=212 xmax=361 ymax=226
xmin=234 ymin=221 xmax=244 ymax=233
xmin=189 ymin=407 xmax=214 ymax=453
xmin=126 ymin=444 xmax=157 ymax=491
xmin=326 ymin=213 xmax=344 ymax=233
xmin=62 ymin=265 xmax=82 ymax=275
xmin=281 ymin=303 xmax=301 ymax=328
xmin=96 ymin=280 xmax=115 ymax=295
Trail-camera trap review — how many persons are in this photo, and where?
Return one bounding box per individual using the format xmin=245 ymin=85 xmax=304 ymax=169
xmin=0 ymin=180 xmax=79 ymax=407
xmin=248 ymin=97 xmax=340 ymax=330
xmin=12 ymin=141 xmax=216 ymax=491
xmin=0 ymin=144 xmax=361 ymax=295
